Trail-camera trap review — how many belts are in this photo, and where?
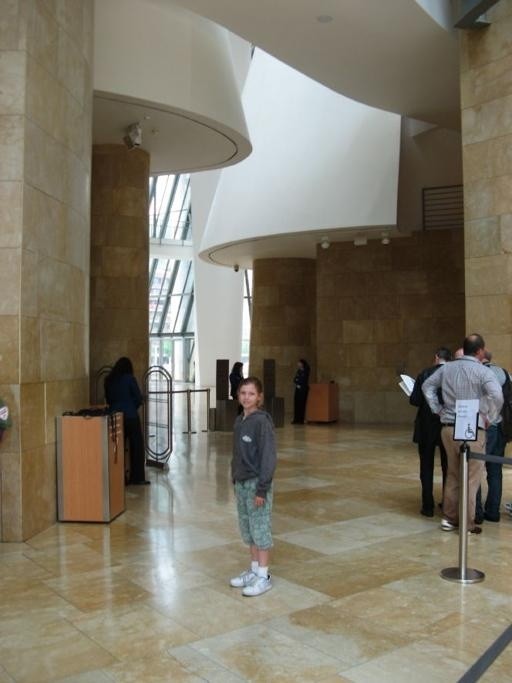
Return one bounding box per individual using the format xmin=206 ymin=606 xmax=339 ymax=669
xmin=441 ymin=422 xmax=483 ymax=429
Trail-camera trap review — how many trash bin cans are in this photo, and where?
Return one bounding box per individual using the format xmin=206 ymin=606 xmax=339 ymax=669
xmin=55 ymin=411 xmax=126 ymax=523
xmin=305 ymin=383 xmax=338 ymax=424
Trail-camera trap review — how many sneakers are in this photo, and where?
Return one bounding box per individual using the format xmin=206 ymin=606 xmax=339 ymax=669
xmin=230 ymin=568 xmax=257 ymax=586
xmin=472 ymin=526 xmax=481 ymax=533
xmin=441 ymin=518 xmax=458 ymax=531
xmin=242 ymin=574 xmax=272 ymax=595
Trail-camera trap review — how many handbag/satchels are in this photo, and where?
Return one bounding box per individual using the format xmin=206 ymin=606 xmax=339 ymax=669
xmin=500 ymin=368 xmax=512 ymax=441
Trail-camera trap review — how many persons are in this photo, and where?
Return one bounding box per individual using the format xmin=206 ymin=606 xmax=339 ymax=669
xmin=290 ymin=357 xmax=313 ymax=426
xmin=229 ymin=361 xmax=247 ymax=416
xmin=102 ymin=354 xmax=153 ymax=487
xmin=228 ymin=375 xmax=280 ymax=598
xmin=408 ymin=333 xmax=511 ymax=535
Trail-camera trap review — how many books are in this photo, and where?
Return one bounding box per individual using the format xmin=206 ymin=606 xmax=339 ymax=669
xmin=397 ymin=374 xmax=415 ymax=397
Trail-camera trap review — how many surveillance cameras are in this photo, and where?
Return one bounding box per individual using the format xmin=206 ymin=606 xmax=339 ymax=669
xmin=234 ymin=264 xmax=239 ymax=272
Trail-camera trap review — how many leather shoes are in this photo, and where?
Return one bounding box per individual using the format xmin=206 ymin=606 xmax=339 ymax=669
xmin=129 ymin=479 xmax=150 ymax=484
